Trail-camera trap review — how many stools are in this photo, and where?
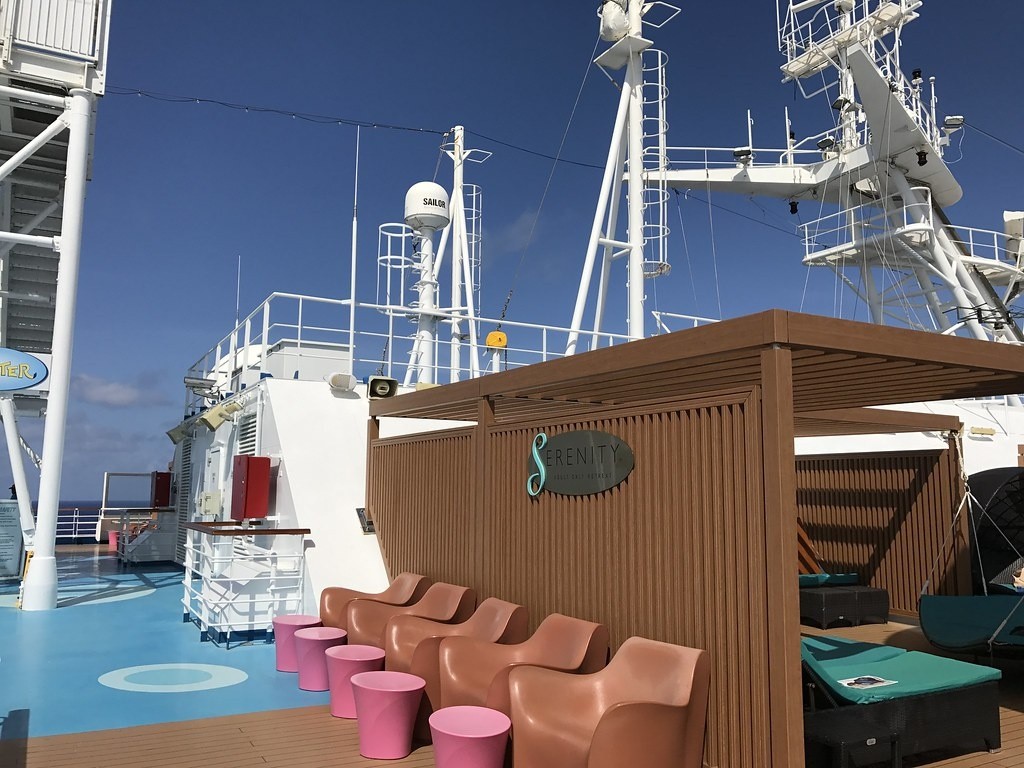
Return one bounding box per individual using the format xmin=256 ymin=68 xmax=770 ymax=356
xmin=799 ymin=587 xmax=855 ymax=630
xmin=294 ymin=626 xmax=348 ymax=693
xmin=325 ymin=644 xmax=385 ymax=720
xmin=350 ymin=671 xmax=426 ymax=760
xmin=427 ymin=706 xmax=511 ymax=768
xmin=108 ymin=531 xmax=120 ymax=546
xmin=273 ymin=615 xmax=321 ymax=672
xmin=840 ymin=586 xmax=890 ymax=626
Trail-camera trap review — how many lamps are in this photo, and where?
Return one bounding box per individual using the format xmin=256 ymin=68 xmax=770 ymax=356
xmin=831 ymin=94 xmax=849 ymax=110
xmin=166 ymin=423 xmax=193 ymax=445
xmin=224 ymin=401 xmax=245 ymax=414
xmin=366 ymin=374 xmax=398 ymax=401
xmin=944 ymin=116 xmax=964 ymax=125
xmin=816 ymin=136 xmax=835 ymax=148
xmin=733 ymin=146 xmax=752 ymax=157
xmin=201 ymin=403 xmax=235 ymax=433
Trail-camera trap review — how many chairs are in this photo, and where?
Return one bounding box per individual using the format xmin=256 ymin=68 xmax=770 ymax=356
xmin=508 ymin=636 xmax=710 ymax=768
xmin=383 ymin=597 xmax=529 ymax=744
xmin=439 ymin=611 xmax=611 ymax=722
xmin=318 ymin=572 xmax=432 ymax=631
xmin=799 ymin=637 xmax=904 ymax=666
xmin=346 ymin=581 xmax=476 ymax=673
xmin=800 ymin=640 xmax=1003 ymax=768
